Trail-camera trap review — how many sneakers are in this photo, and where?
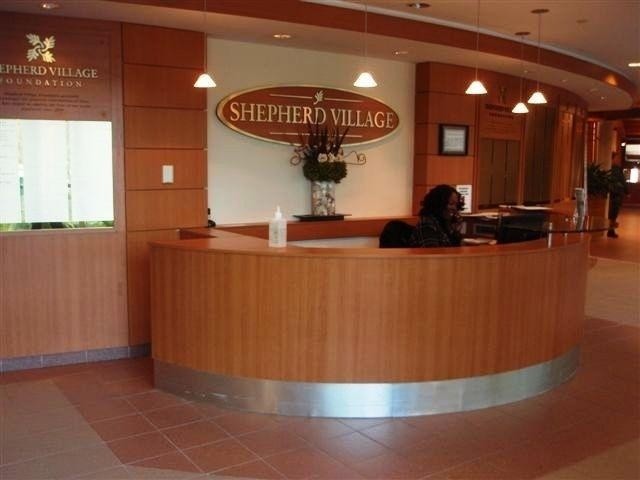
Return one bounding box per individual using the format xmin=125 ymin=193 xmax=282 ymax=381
xmin=607 ymin=230 xmax=618 ymax=238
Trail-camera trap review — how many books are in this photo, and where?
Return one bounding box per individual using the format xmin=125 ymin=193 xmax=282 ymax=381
xmin=498 ymin=203 xmax=554 ymax=211
xmin=460 ymin=211 xmax=509 ymax=222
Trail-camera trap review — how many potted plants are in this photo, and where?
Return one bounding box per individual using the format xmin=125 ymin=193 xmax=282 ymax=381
xmin=587 ymin=162 xmax=611 ymax=216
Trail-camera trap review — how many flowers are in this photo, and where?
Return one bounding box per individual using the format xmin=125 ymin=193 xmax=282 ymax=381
xmin=301 ymin=117 xmax=350 ymax=184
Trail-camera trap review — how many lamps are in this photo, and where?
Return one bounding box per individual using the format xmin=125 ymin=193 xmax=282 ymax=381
xmin=465 ymin=0 xmax=550 ymax=114
xmin=353 ymin=0 xmax=378 ymax=89
xmin=193 ymin=0 xmax=217 ymax=89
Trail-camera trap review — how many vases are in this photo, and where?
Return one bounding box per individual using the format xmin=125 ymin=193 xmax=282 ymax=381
xmin=310 ymin=179 xmax=335 ymax=217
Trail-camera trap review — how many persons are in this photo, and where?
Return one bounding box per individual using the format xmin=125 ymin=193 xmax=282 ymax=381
xmin=607 ymin=152 xmax=631 ymax=238
xmin=406 ymin=183 xmax=467 ymax=249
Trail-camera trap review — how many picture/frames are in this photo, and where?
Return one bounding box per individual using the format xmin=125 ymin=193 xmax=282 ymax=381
xmin=438 ymin=123 xmax=470 ymax=157
